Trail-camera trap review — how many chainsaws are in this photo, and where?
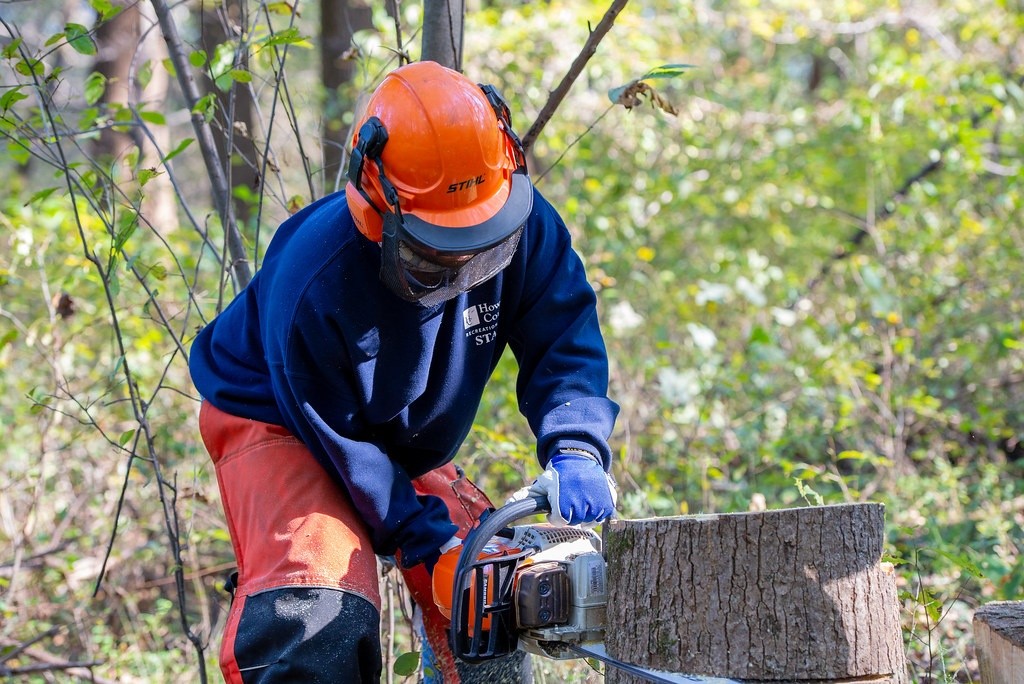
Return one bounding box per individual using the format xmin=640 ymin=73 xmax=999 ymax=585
xmin=430 ymin=496 xmax=745 ymax=684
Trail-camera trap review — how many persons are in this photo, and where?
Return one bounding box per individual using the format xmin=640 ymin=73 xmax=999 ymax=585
xmin=189 ymin=60 xmax=620 ymax=684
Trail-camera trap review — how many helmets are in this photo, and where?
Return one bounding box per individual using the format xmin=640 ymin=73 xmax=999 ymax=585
xmin=347 ymin=60 xmax=534 ymax=247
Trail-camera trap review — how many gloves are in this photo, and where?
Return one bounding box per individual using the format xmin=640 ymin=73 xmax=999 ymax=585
xmin=424 ymin=541 xmax=502 ymax=605
xmin=504 ymin=448 xmax=614 ymax=525
